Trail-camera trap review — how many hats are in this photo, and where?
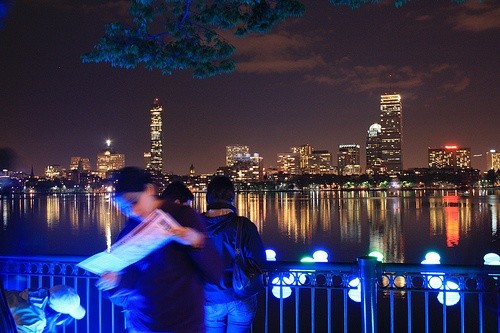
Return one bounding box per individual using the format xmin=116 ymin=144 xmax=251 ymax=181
xmin=111 ymin=166 xmax=151 ymax=192
xmin=206 ymin=174 xmax=235 ymax=192
xmin=47 ymin=285 xmax=86 ymax=320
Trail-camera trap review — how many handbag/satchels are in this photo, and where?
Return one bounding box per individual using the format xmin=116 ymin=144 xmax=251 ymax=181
xmin=233 ymin=217 xmax=266 ymax=302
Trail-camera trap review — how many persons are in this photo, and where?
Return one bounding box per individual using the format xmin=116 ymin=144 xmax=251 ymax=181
xmin=4 ymin=284 xmax=86 ymax=333
xmin=198 ymin=176 xmax=267 ymax=333
xmin=96 ymin=166 xmax=225 ymax=333
xmin=159 ymin=179 xmax=196 ymax=207
